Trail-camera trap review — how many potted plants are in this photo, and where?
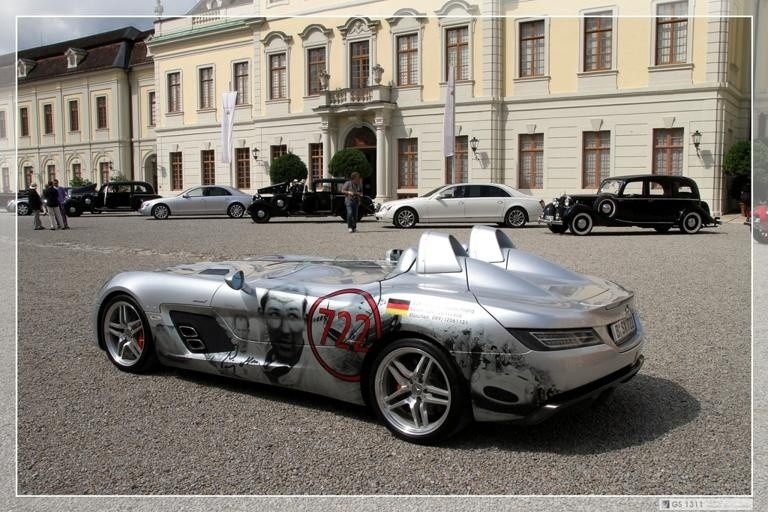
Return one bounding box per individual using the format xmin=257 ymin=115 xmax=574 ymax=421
xmin=724 ymin=138 xmax=768 ymax=218
xmin=372 ymin=63 xmax=385 ymax=85
xmin=319 ymin=70 xmax=330 ymax=89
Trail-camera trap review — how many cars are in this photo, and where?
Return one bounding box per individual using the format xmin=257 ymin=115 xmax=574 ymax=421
xmin=538 ymin=174 xmax=720 ymax=234
xmin=250 ymin=179 xmax=375 ymax=224
xmin=374 ymin=182 xmax=544 ymax=227
xmin=139 ymin=184 xmax=255 ymax=219
xmin=8 ymin=181 xmax=161 ymax=217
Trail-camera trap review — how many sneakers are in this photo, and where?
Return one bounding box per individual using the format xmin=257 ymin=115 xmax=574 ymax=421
xmin=349 ymin=228 xmax=356 ymax=232
xmin=34 ymin=226 xmax=45 ymax=230
xmin=51 ymin=225 xmax=69 ymax=231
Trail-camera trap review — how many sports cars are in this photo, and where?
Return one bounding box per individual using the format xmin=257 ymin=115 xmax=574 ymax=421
xmin=93 ymin=225 xmax=646 ymax=446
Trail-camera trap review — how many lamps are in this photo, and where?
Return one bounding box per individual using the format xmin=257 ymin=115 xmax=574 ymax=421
xmin=251 ymin=148 xmax=260 ymax=160
xmin=691 ymin=131 xmax=702 ymax=149
xmin=469 ymin=137 xmax=480 ymax=155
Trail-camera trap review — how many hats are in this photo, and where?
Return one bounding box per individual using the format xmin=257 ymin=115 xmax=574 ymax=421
xmin=30 ymin=182 xmax=39 ymax=189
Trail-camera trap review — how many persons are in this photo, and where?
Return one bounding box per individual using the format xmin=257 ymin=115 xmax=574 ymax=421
xmin=46 ymin=181 xmax=67 ymax=230
xmin=440 ymin=328 xmax=561 ymax=405
xmin=341 ymin=172 xmax=363 ymax=232
xmin=260 ymin=280 xmax=341 ymax=398
xmin=52 ymin=178 xmax=70 ymax=229
xmin=27 ymin=183 xmax=45 ymax=230
xmin=231 ymin=313 xmax=251 ymax=355
xmin=42 ymin=184 xmax=48 ymax=196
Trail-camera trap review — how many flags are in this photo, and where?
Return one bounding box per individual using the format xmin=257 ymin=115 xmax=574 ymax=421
xmin=443 ymin=63 xmax=455 ymax=159
xmin=219 ymin=90 xmax=238 ymax=166
